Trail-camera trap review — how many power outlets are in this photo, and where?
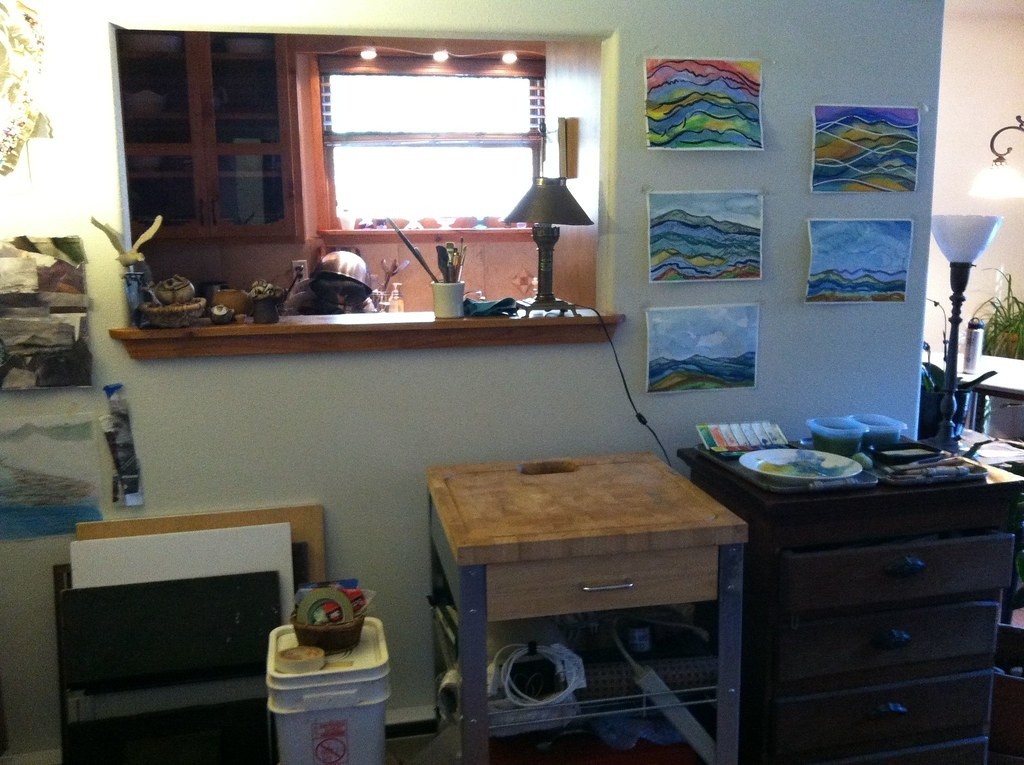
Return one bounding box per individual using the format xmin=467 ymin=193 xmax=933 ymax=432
xmin=293 ymin=260 xmax=308 ymax=281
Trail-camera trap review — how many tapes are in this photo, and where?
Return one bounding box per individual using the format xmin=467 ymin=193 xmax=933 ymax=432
xmin=275 ymin=646 xmax=325 ymax=674
xmin=296 ymin=587 xmax=353 ymax=625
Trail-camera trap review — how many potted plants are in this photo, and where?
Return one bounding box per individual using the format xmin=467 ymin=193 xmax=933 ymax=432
xmin=972 ymin=267 xmax=1024 ymax=360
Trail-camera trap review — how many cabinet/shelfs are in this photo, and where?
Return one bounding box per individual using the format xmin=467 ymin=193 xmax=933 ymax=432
xmin=116 ymin=31 xmax=316 ymax=245
xmin=677 ymin=438 xmax=1024 ymax=765
xmin=428 ymin=455 xmax=749 ymax=765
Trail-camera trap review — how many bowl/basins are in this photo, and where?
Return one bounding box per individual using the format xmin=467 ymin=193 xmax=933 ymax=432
xmin=309 ymin=251 xmax=372 ymax=296
xmin=804 ymin=416 xmax=869 ymax=456
xmin=848 ymin=413 xmax=908 ymax=448
xmin=291 ymin=615 xmax=364 ymax=651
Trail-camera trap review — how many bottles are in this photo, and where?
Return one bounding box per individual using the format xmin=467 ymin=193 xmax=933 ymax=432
xmin=963 ymin=318 xmax=985 ymax=375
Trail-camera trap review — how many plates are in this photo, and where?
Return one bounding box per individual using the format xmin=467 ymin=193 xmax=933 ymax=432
xmin=738 ymin=448 xmax=864 ymax=485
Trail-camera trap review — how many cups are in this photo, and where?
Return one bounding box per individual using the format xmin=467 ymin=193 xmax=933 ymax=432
xmin=430 ymin=280 xmax=465 ymax=318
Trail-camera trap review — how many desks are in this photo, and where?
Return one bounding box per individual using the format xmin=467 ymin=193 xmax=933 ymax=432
xmin=922 ymin=350 xmax=1024 ymax=439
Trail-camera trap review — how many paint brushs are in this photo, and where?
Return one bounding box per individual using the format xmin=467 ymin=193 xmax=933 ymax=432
xmin=893 ymin=465 xmax=975 ymax=475
xmin=446 ymin=243 xmax=454 ymax=283
xmin=388 ymin=217 xmax=439 ymax=283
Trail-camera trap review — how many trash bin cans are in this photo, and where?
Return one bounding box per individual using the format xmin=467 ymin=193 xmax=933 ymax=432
xmin=993 ymin=624 xmax=1023 ymax=755
xmin=266 ymin=616 xmax=390 ymax=764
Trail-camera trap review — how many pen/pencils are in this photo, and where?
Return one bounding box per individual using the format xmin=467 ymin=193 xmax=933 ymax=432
xmin=453 ymin=237 xmax=467 ymax=283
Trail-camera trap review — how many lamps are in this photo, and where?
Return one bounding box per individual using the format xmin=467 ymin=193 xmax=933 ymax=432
xmin=969 ymin=116 xmax=1024 ymax=202
xmin=508 ymin=178 xmax=598 ymax=319
xmin=932 ymin=215 xmax=1002 ymax=439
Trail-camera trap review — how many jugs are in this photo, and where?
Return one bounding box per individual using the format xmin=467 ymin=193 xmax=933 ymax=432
xmin=154 ymin=275 xmax=196 ymax=305
